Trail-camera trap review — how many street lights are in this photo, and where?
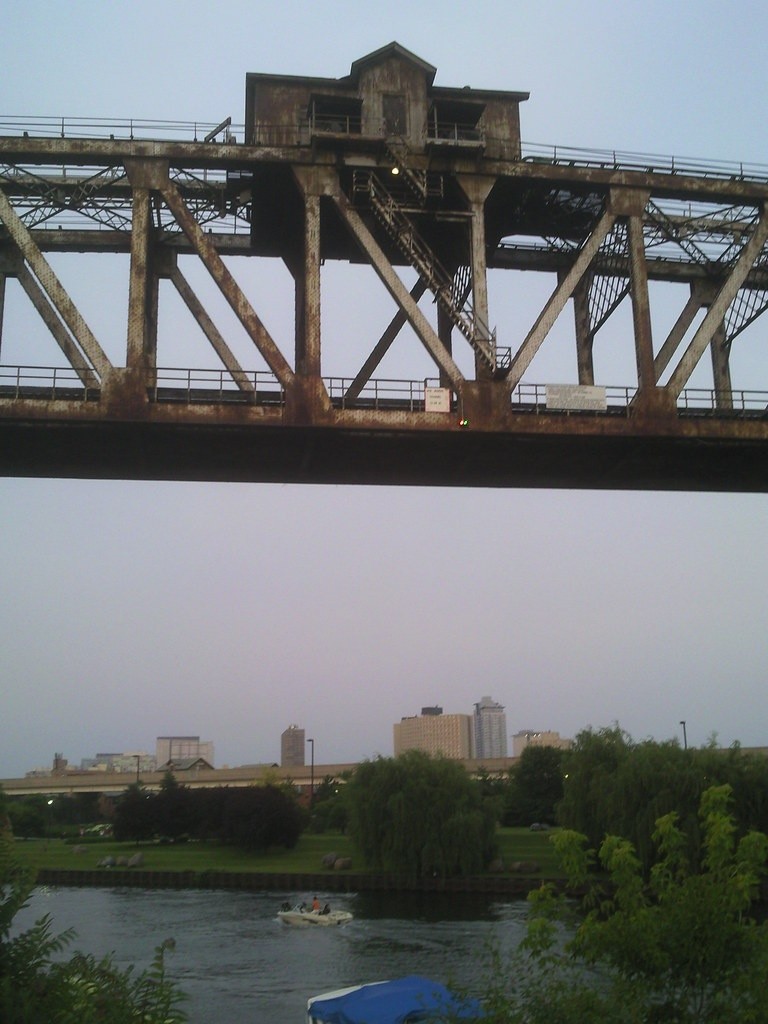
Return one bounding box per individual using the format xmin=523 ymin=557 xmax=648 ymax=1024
xmin=306 ymin=738 xmax=314 ymax=795
xmin=131 ymin=755 xmax=140 ymax=783
xmin=679 ymin=720 xmax=687 ymax=750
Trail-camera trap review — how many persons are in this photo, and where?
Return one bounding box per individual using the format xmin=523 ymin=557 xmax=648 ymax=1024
xmin=291 ymin=901 xmax=307 ymax=913
xmin=281 ymin=902 xmax=290 ymax=911
xmin=320 ymin=904 xmax=330 ymax=915
xmin=311 ymin=897 xmax=320 ymax=915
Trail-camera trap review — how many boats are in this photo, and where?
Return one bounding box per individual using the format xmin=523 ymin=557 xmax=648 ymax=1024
xmin=305 ymin=976 xmax=490 ymax=1024
xmin=276 ymin=902 xmax=353 ymax=925
